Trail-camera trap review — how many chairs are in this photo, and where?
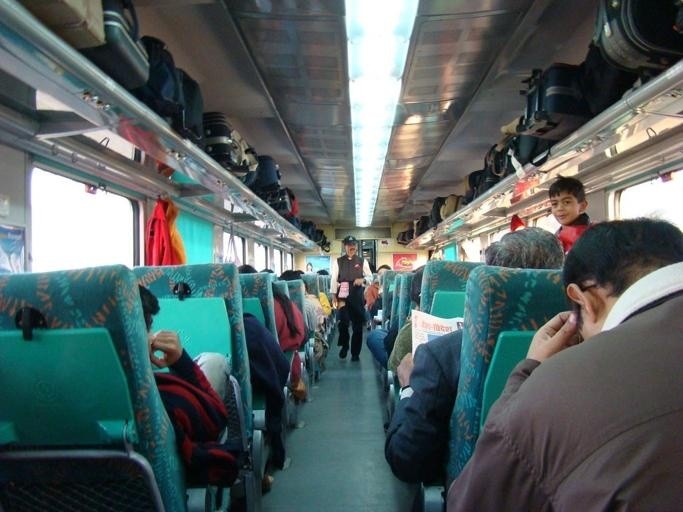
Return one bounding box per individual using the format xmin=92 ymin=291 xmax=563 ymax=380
xmin=0 ymin=264 xmax=340 ymax=507
xmin=371 ymin=259 xmax=585 ymax=512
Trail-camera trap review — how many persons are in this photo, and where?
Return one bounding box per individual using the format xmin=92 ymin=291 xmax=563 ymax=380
xmin=331 ymin=235 xmax=373 ymax=361
xmin=260 ymin=269 xmax=329 ymax=381
xmin=138 ymin=283 xmax=237 ymax=499
xmin=548 ymin=175 xmax=597 ymax=258
xmin=381 ymin=224 xmax=566 ymax=511
xmin=445 ymin=216 xmax=683 ymax=511
xmin=243 ymin=311 xmax=290 ymax=491
xmin=238 ymin=265 xmax=306 ymax=404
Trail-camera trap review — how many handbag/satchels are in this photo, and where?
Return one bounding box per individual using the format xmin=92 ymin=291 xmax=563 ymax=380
xmin=292 ymin=380 xmax=307 ymax=399
xmin=396 ymin=116 xmax=554 ymax=249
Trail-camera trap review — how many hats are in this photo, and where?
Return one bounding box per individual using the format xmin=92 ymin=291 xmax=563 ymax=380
xmin=343 ymin=236 xmax=358 ymax=245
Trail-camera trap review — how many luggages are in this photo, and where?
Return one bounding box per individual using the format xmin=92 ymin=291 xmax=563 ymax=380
xmin=517 ymin=59 xmax=592 ymax=141
xmin=25 ymin=0 xmax=105 ymax=51
xmin=133 ymin=36 xmax=184 ymax=117
xmin=595 ymin=0 xmax=683 ymax=77
xmin=176 ymin=65 xmax=335 ymax=254
xmin=88 ymin=0 xmax=152 ymax=91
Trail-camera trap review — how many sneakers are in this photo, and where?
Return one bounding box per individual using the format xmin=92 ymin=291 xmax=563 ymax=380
xmin=339 ymin=345 xmax=359 ymax=361
